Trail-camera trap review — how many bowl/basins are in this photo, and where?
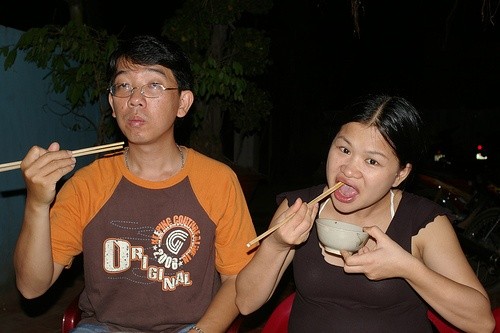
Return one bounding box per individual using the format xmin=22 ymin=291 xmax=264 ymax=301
xmin=315 ymin=217 xmax=369 ymax=256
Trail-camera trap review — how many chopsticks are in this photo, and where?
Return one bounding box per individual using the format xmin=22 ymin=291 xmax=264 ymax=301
xmin=0 ymin=142 xmax=125 ymax=173
xmin=246 ymin=181 xmax=346 ymax=247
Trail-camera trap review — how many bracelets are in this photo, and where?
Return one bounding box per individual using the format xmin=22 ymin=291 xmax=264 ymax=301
xmin=192 ymin=325 xmax=203 ymax=333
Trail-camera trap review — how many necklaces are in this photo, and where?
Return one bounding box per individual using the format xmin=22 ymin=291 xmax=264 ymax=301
xmin=125 ymin=144 xmax=184 ymax=170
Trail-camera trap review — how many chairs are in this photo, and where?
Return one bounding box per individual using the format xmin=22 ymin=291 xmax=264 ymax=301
xmin=261 ymin=291 xmax=463 ymax=333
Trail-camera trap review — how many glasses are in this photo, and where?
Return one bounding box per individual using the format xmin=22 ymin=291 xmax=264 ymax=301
xmin=106 ymin=81 xmax=179 ymax=99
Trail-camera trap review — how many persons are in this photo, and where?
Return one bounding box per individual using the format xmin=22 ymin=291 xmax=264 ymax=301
xmin=235 ymin=91 xmax=495 ymax=333
xmin=14 ymin=35 xmax=260 ymax=333
